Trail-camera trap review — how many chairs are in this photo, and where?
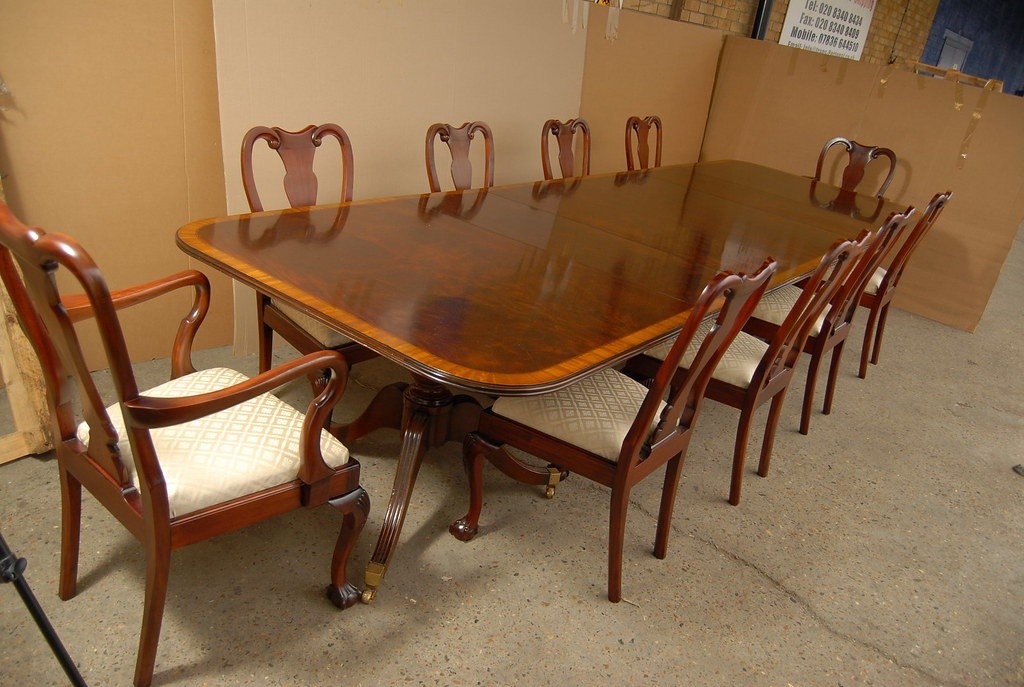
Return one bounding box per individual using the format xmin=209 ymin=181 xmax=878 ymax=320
xmin=446 ymin=250 xmax=781 ymax=604
xmin=815 ymin=133 xmax=897 ymax=199
xmin=622 ymin=113 xmax=665 ymax=167
xmin=796 ymin=189 xmax=960 ymax=378
xmin=742 ymin=204 xmax=918 ymax=436
xmin=540 ymin=114 xmax=593 ymax=180
xmin=0 ymin=186 xmax=369 ymax=687
xmin=240 ymin=122 xmax=377 ymax=430
xmin=423 ymin=118 xmax=494 ymax=191
xmin=628 ymin=218 xmax=875 ymax=510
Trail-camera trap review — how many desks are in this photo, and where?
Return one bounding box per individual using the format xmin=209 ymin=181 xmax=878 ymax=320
xmin=169 ymin=151 xmax=925 ymax=606
